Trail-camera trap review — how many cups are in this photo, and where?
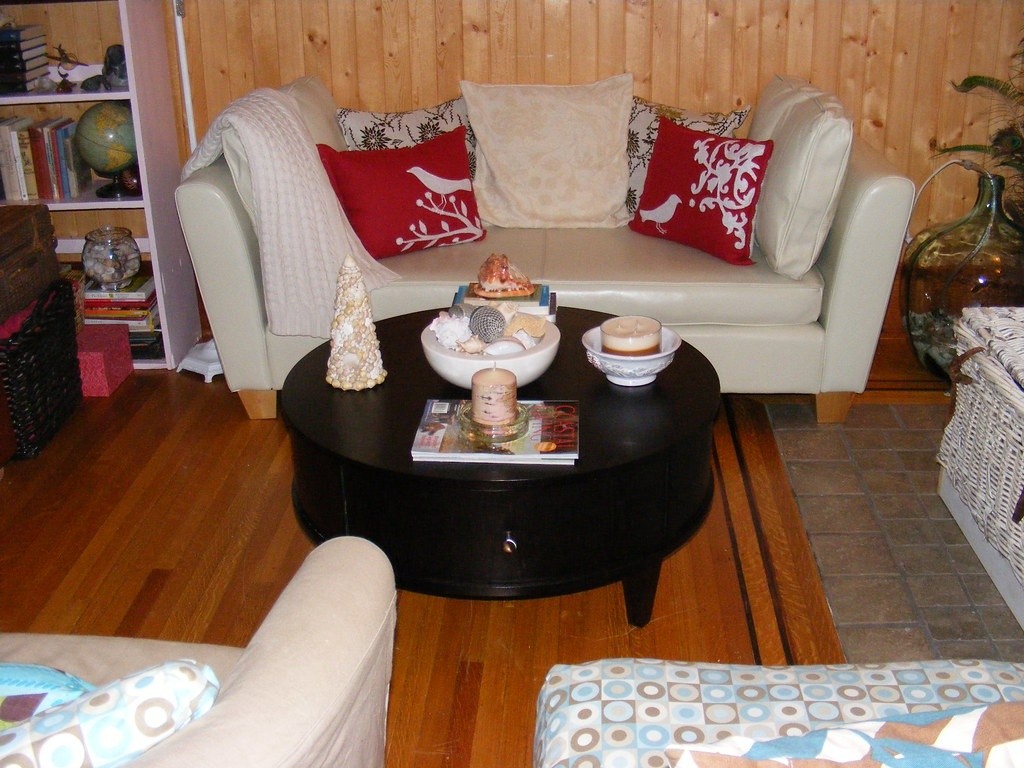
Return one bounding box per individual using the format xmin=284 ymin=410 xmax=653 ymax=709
xmin=599 ymin=315 xmax=662 ymax=356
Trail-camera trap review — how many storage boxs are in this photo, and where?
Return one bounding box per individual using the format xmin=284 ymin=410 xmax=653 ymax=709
xmin=75 ymin=324 xmax=132 ymax=398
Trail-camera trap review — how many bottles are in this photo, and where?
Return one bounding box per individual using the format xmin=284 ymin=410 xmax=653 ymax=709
xmin=82 ymin=227 xmax=142 ymax=289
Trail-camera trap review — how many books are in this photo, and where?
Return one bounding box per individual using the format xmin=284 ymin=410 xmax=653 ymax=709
xmin=0 ymin=115 xmax=92 ymax=202
xmin=0 ymin=23 xmax=51 ymax=92
xmin=83 ymin=276 xmax=161 ymax=347
xmin=411 ymin=398 xmax=581 ymax=466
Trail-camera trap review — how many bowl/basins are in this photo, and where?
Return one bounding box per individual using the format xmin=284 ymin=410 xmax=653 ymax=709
xmin=582 ymin=327 xmax=682 ymax=387
xmin=421 ymin=312 xmax=561 ymax=389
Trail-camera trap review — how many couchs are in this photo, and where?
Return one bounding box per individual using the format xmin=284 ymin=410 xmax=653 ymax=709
xmin=175 ymin=76 xmax=917 ymax=421
xmin=534 ymin=659 xmax=1024 ymax=768
xmin=1 ymin=535 xmax=394 ymax=767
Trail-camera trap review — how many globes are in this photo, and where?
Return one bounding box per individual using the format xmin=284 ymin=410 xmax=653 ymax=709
xmin=75 ymin=102 xmax=141 ymax=198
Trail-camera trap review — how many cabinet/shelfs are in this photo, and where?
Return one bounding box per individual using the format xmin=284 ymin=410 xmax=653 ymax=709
xmin=0 ymin=0 xmax=202 ymax=370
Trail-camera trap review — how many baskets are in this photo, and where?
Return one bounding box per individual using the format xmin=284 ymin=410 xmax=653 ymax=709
xmin=934 ymin=306 xmax=1024 ymax=582
xmin=0 ymin=279 xmax=84 ymax=458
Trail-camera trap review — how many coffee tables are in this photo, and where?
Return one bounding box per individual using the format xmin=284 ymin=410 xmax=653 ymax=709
xmin=278 ymin=299 xmax=721 ymax=629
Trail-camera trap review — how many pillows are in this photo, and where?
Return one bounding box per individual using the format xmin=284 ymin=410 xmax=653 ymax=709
xmin=628 ymin=117 xmax=774 ymax=266
xmin=316 ymin=125 xmax=487 ymax=259
xmin=740 ymin=76 xmax=856 ymax=278
xmin=627 ymin=95 xmax=752 ymax=212
xmin=0 ymin=657 xmax=221 ymax=767
xmin=460 ymin=69 xmax=636 ymax=229
xmin=221 ymin=73 xmax=352 ymax=239
xmin=336 ymin=95 xmax=478 ymax=183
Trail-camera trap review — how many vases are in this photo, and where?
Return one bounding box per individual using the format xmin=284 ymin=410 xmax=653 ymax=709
xmin=82 ymin=228 xmax=143 ymax=291
